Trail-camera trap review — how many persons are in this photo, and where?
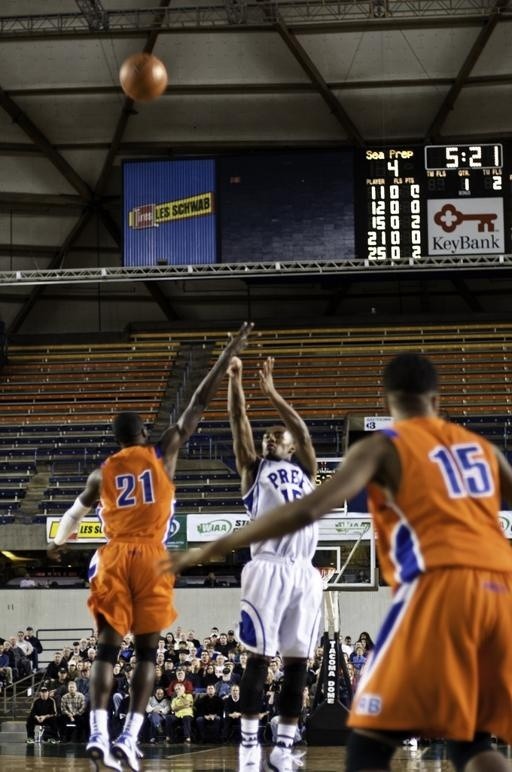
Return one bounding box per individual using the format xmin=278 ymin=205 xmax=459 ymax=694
xmin=158 ymin=351 xmax=512 ymax=771
xmin=356 ymin=566 xmax=370 ymax=582
xmin=205 ymin=571 xmax=217 ymax=587
xmin=46 ymin=320 xmax=256 ymax=772
xmin=20 ymin=573 xmax=36 ymax=590
xmin=226 ymin=354 xmax=323 ymax=772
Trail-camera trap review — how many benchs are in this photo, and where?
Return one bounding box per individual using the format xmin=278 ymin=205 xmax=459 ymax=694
xmin=0 ymin=330 xmax=512 ymax=525
xmin=291 ymin=323 xmax=512 ymax=458
xmin=0 ymin=331 xmax=183 ymax=525
xmin=184 ymin=331 xmax=291 ymax=514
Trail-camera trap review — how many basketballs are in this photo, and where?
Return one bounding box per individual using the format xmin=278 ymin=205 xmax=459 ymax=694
xmin=120 ymin=55 xmax=168 ymax=100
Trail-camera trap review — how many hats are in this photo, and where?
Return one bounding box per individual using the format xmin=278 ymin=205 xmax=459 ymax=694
xmin=40 ymin=686 xmax=48 ymax=692
xmin=58 ymin=668 xmax=67 ymax=673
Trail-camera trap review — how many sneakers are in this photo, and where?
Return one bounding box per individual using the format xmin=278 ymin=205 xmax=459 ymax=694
xmin=84 ymin=734 xmax=122 ymax=772
xmin=25 ymin=738 xmax=60 ymax=744
xmin=238 ymin=742 xmax=262 ymax=771
xmin=267 ymin=746 xmax=294 ymax=771
xmin=151 ymin=736 xmax=191 ymax=743
xmin=110 ymin=732 xmax=140 ymax=771
xmin=408 ymin=738 xmax=418 ymax=746
